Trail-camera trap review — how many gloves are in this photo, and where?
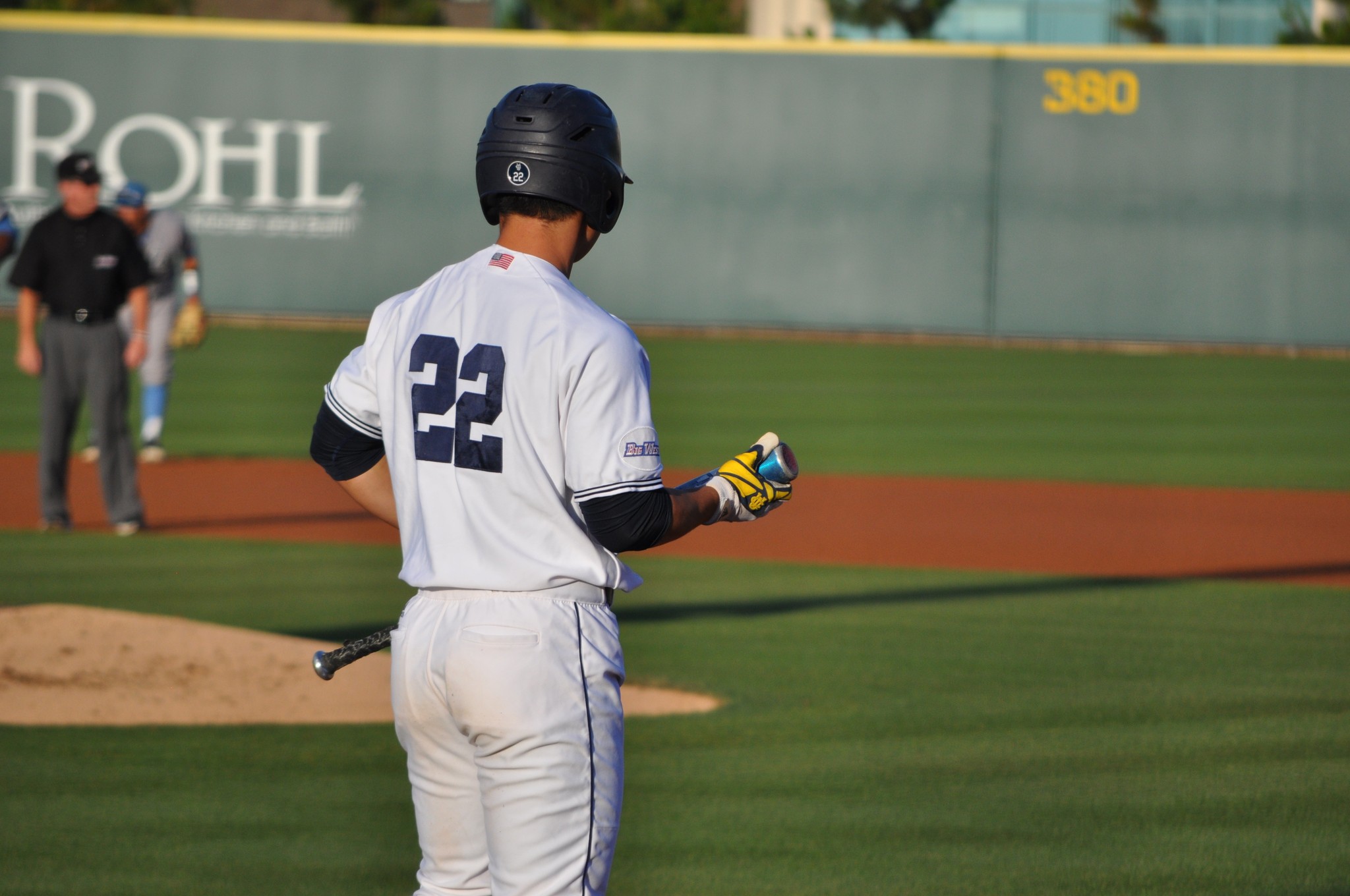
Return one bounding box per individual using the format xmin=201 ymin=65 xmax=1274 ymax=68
xmin=706 ymin=432 xmax=793 ymax=525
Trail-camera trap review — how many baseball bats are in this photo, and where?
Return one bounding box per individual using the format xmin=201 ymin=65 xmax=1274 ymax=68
xmin=310 ymin=443 xmax=796 ymax=682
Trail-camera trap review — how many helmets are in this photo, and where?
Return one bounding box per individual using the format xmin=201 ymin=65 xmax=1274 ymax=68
xmin=475 ymin=82 xmax=633 ymax=234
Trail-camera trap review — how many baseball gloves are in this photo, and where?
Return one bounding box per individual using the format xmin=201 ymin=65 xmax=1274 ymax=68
xmin=168 ymin=298 xmax=213 ymax=347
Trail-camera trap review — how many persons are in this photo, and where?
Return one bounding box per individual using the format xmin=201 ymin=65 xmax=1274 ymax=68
xmin=8 ymin=149 xmax=208 ymax=538
xmin=308 ymin=83 xmax=796 ymax=896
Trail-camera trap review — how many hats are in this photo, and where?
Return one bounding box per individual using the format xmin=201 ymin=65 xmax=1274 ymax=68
xmin=116 ymin=181 xmax=145 ymax=207
xmin=56 ymin=154 xmax=98 ymax=184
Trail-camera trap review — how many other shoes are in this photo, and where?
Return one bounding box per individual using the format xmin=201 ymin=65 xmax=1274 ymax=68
xmin=141 ymin=444 xmax=164 ymax=464
xmin=115 ymin=519 xmax=138 ymax=538
xmin=86 ymin=444 xmax=102 ymax=462
